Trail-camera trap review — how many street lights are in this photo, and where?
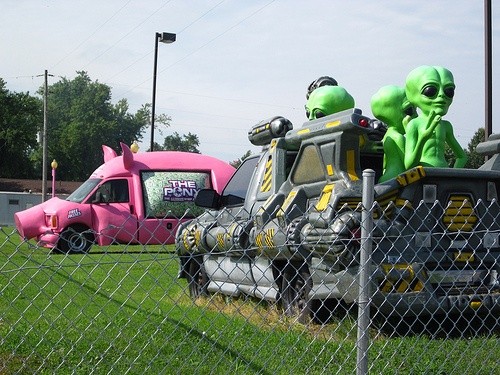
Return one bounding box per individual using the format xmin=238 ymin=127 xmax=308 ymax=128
xmin=150 ymin=31 xmax=177 ymax=153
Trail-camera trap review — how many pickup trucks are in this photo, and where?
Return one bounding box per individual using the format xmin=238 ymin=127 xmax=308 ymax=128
xmin=174 ymin=107 xmax=500 ymax=324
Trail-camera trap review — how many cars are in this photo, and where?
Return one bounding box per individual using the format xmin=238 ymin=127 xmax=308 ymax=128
xmin=12 ymin=141 xmax=237 ymax=255
